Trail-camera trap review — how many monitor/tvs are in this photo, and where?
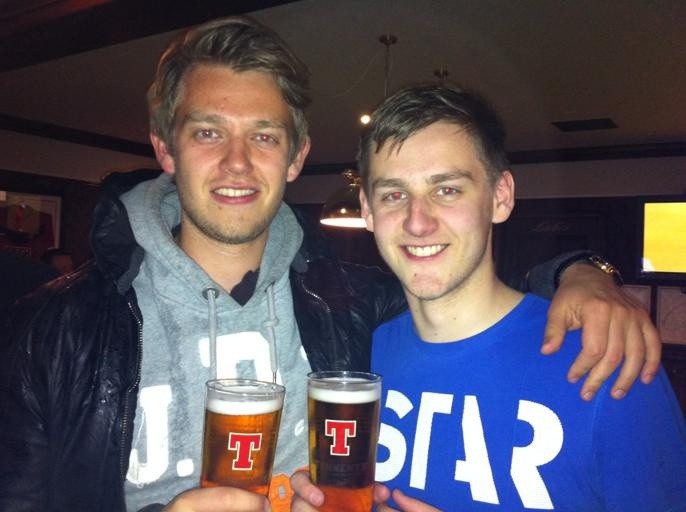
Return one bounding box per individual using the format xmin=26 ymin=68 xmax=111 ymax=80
xmin=633 ymin=195 xmax=686 ymax=279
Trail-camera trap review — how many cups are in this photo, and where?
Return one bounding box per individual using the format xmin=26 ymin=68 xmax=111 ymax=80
xmin=306 ymin=366 xmax=381 ymax=512
xmin=199 ymin=378 xmax=285 ymax=497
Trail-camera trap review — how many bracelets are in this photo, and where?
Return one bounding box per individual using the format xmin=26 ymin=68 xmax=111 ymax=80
xmin=557 ymin=254 xmax=625 ymax=289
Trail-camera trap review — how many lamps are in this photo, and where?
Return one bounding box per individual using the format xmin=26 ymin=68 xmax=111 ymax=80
xmin=320 ymin=34 xmax=399 ymax=230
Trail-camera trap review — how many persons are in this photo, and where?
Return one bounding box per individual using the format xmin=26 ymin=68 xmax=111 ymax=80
xmin=289 ymin=85 xmax=684 ymax=512
xmin=1 ymin=17 xmax=663 ymax=511
